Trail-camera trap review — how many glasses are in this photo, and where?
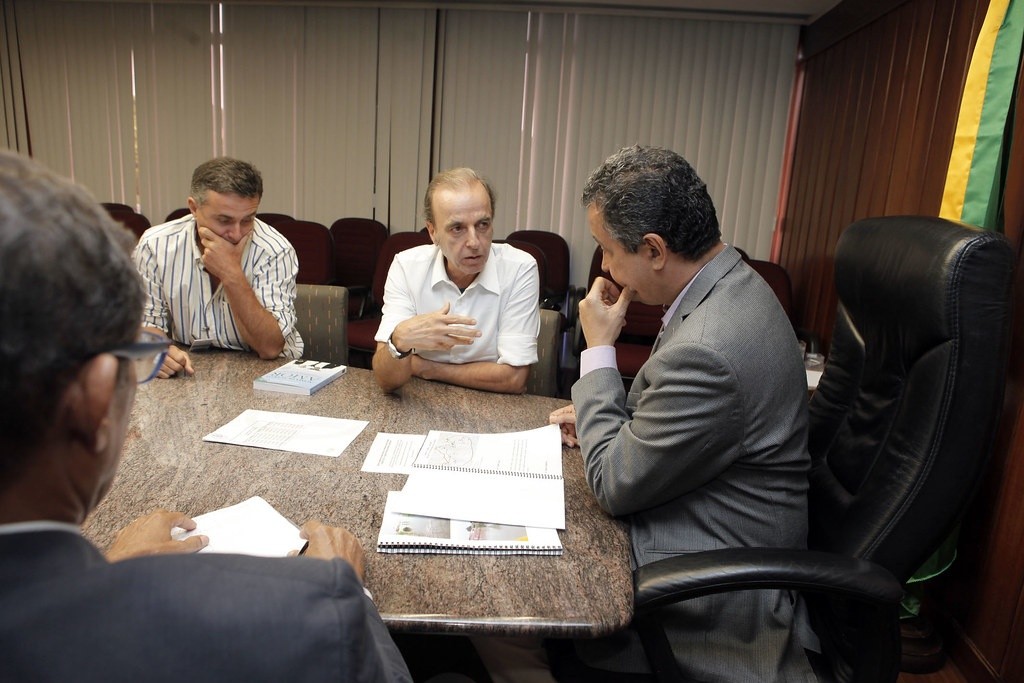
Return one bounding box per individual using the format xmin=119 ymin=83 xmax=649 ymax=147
xmin=109 ymin=330 xmax=169 ymax=387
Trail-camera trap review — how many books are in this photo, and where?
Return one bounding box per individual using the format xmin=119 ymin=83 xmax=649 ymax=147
xmin=392 ymin=423 xmax=565 ymax=530
xmin=253 ymin=358 xmax=347 ymax=396
xmin=377 ymin=490 xmax=563 ymax=557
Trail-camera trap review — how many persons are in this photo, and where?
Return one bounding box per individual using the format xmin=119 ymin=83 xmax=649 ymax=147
xmin=372 ymin=166 xmax=540 ymax=394
xmin=471 ymin=145 xmax=822 ymax=683
xmin=0 ymin=149 xmax=413 ymax=683
xmin=131 ymin=157 xmax=304 ymax=379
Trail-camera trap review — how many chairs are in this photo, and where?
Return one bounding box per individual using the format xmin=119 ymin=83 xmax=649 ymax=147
xmin=544 ymin=215 xmax=1017 ymax=683
xmin=95 ymin=202 xmax=794 ymax=400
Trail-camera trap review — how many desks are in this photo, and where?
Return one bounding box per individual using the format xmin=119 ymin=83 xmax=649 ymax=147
xmin=80 ymin=350 xmax=635 ymax=632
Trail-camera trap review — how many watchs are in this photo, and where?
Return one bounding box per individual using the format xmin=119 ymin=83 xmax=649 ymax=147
xmin=387 ymin=333 xmax=415 ymax=360
